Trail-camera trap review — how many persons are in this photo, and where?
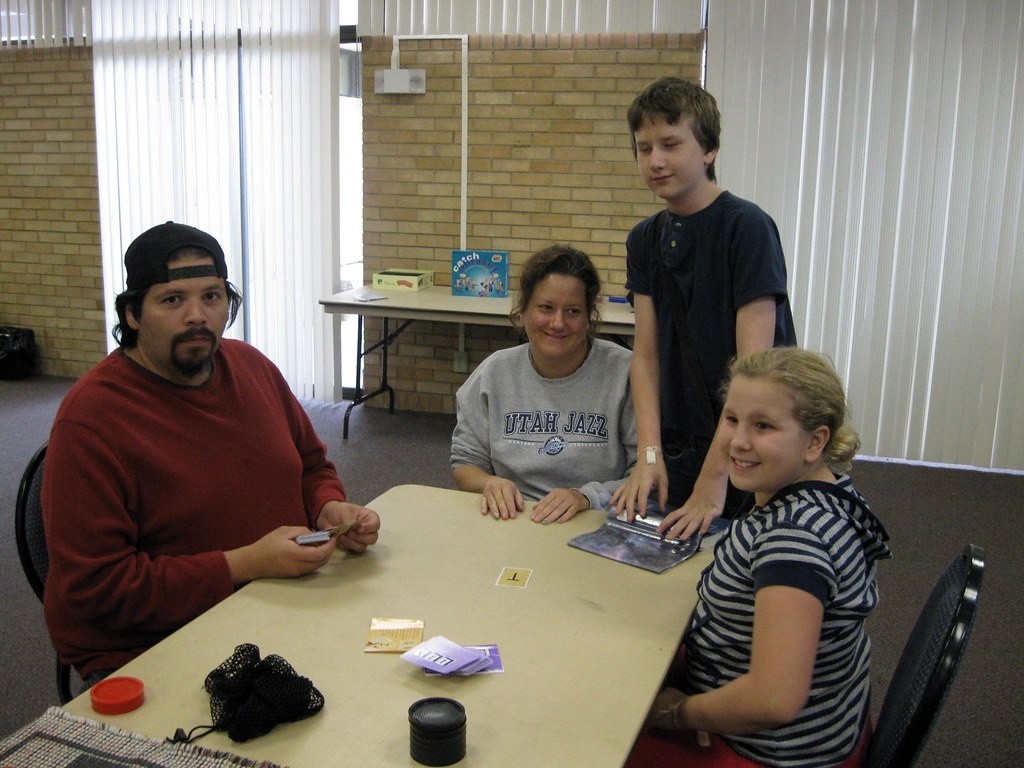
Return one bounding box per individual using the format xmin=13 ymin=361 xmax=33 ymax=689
xmin=610 ymin=343 xmax=891 ymax=768
xmin=46 ymin=220 xmax=382 ymax=694
xmin=608 ymin=75 xmax=799 ymax=545
xmin=449 ymin=243 xmax=667 ymax=525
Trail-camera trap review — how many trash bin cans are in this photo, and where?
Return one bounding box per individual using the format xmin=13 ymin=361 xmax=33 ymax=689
xmin=1 ymin=325 xmax=35 ymax=381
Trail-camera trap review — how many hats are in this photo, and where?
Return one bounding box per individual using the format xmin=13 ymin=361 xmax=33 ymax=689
xmin=124 ymin=220 xmax=228 ymax=286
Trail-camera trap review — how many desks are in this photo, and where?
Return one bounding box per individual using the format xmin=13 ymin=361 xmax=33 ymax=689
xmin=317 ymin=284 xmax=636 ymax=441
xmin=1 ymin=482 xmax=735 ymax=768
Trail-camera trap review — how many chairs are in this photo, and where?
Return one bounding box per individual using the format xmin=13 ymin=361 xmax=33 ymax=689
xmin=857 ymin=542 xmax=987 ymax=767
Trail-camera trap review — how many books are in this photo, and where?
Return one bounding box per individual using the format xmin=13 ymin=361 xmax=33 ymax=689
xmin=568 ymin=493 xmax=732 ymax=575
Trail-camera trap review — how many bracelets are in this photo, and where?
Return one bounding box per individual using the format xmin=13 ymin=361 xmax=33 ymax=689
xmin=659 ymin=691 xmax=689 ymax=730
xmin=636 ymin=445 xmax=664 ymax=465
